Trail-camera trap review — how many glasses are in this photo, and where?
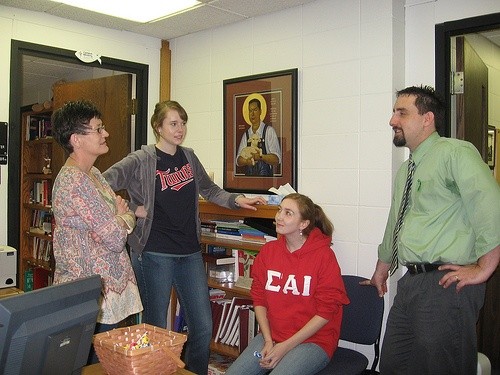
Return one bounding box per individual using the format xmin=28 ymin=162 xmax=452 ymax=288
xmin=80 ymin=124 xmax=105 ymax=135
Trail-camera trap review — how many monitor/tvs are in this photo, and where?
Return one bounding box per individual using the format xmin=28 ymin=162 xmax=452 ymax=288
xmin=0 ymin=274 xmax=102 ymax=375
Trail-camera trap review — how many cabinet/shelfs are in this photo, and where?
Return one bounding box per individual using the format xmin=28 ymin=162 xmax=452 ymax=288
xmin=18 ymin=106 xmax=54 ymax=290
xmin=171 ymin=203 xmax=280 ymax=358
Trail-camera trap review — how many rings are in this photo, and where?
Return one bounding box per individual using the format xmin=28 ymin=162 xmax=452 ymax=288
xmin=455 ymin=274 xmax=458 ymax=282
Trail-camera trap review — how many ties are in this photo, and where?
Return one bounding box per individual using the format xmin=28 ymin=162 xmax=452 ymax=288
xmin=388 ymin=154 xmax=415 ymax=278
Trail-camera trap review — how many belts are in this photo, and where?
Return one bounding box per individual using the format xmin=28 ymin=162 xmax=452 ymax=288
xmin=406 ymin=262 xmax=446 ymax=276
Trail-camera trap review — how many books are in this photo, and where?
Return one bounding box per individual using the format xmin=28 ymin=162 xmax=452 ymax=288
xmin=203 ymin=243 xmax=259 ymax=290
xmin=175 ymin=287 xmax=259 ymax=353
xmin=21 ymin=266 xmax=56 ymax=293
xmin=200 ymin=213 xmax=279 ymax=244
xmin=28 ymin=179 xmax=53 ymax=206
xmin=29 ymin=209 xmax=54 ymax=234
xmin=30 ymin=236 xmax=54 ymax=263
xmin=26 ymin=115 xmax=52 ymax=140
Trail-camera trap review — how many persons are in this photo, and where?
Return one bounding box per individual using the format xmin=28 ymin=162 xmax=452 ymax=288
xmin=224 ymin=193 xmax=350 ymax=375
xmin=50 ymin=101 xmax=144 ymax=366
xmin=101 ymin=100 xmax=267 ymax=375
xmin=359 ymin=86 xmax=500 ymax=375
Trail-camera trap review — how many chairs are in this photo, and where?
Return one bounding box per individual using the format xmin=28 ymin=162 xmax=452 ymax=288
xmin=314 ymin=275 xmax=384 ymax=375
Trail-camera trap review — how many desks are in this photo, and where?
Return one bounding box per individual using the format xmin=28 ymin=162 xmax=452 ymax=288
xmin=0 ymin=287 xmax=24 ymax=298
xmin=82 ymin=360 xmax=195 ymax=375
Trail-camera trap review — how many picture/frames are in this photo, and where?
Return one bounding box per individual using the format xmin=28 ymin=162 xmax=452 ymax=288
xmin=223 ymin=67 xmax=298 ymax=195
xmin=487 ymin=127 xmax=496 ymax=167
